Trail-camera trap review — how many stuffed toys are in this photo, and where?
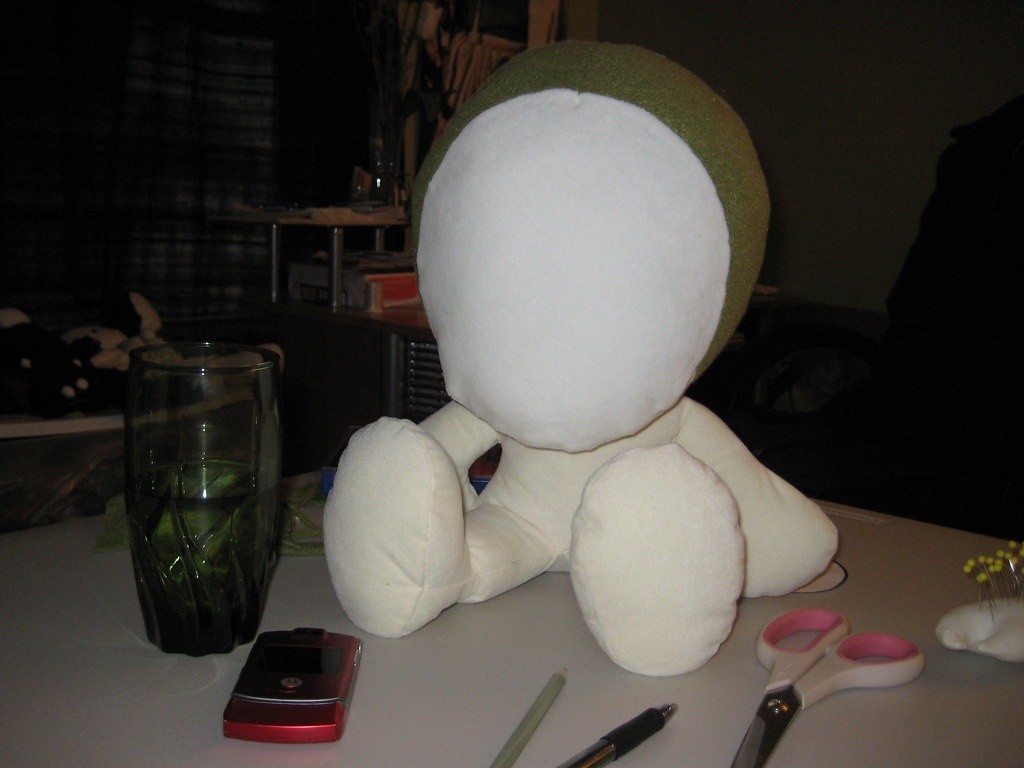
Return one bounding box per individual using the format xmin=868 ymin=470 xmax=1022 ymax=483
xmin=322 ymin=39 xmax=838 ymax=676
xmin=1 ymin=292 xmax=183 ymax=420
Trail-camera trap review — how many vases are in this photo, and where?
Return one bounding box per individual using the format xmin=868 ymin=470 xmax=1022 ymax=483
xmin=368 ymin=112 xmax=406 ymax=206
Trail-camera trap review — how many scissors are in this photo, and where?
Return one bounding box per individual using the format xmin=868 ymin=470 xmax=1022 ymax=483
xmin=729 ymin=608 xmax=925 ymax=768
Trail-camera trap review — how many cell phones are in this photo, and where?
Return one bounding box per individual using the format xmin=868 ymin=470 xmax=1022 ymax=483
xmin=223 ymin=627 xmax=363 ymax=744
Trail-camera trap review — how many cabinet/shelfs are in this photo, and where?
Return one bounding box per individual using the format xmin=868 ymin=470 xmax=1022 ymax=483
xmin=206 ymin=205 xmax=746 ymax=481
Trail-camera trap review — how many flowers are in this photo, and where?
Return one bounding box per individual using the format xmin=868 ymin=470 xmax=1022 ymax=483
xmin=351 ymin=0 xmax=444 ymax=168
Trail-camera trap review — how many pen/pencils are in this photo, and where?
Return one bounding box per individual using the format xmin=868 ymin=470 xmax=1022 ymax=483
xmin=557 ymin=704 xmax=677 ymax=768
xmin=488 ymin=667 xmax=568 ymax=768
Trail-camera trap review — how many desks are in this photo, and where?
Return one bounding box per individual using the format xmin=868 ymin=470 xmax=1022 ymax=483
xmin=0 ymin=469 xmax=1024 ymax=768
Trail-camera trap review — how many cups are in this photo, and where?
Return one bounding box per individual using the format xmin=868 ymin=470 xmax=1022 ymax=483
xmin=122 ymin=338 xmax=286 ymax=655
